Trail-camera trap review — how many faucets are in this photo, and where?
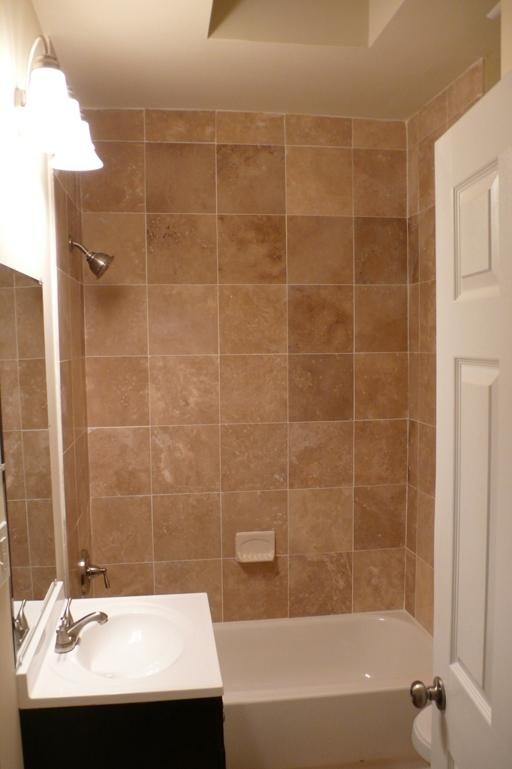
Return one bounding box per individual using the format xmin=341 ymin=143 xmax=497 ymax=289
xmin=54 ymin=596 xmax=110 ymax=652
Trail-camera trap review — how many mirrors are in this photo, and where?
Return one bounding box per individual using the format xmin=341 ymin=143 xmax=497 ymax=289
xmin=0 ymin=260 xmax=64 ymax=693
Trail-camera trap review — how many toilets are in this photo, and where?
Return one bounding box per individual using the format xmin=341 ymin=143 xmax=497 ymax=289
xmin=410 ymin=703 xmax=433 ymax=764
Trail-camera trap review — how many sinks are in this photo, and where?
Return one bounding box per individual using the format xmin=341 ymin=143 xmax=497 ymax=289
xmin=31 ymin=591 xmax=224 ymax=708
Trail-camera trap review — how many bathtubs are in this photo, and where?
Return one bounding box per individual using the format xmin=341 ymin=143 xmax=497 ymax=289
xmin=211 ymin=608 xmax=436 ymax=769
